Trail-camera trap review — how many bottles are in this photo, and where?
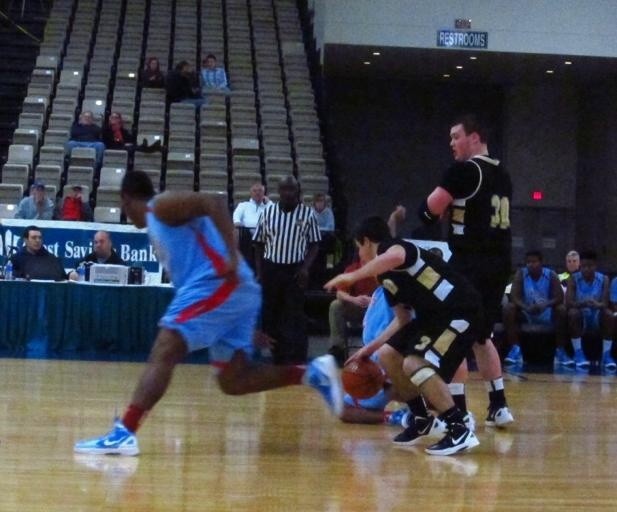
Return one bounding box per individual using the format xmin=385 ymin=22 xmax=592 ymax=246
xmin=77 ymin=261 xmax=86 ymax=282
xmin=4 ymin=260 xmax=13 ymax=279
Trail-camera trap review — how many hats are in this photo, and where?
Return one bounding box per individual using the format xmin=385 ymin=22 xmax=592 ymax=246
xmin=71 ymin=183 xmax=82 ymax=191
xmin=30 ymin=180 xmax=47 ymax=190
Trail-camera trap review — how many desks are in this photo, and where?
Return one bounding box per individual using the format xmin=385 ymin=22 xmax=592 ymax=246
xmin=0 ymin=278 xmax=209 ymax=364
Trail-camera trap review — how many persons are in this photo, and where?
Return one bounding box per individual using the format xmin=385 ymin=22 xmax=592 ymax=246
xmin=328 ymin=260 xmax=382 ymax=368
xmin=106 ymin=111 xmax=162 ymax=153
xmin=555 ymin=250 xmax=581 ymax=357
xmin=67 ymin=230 xmax=127 ymax=282
xmin=233 ymin=183 xmax=274 ymax=278
xmin=324 ymin=216 xmax=489 ymax=454
xmin=603 ymin=277 xmax=616 ymax=367
xmin=13 ymin=181 xmax=56 ymax=220
xmin=51 ymin=186 xmax=95 ymax=222
xmin=304 ymin=205 xmax=444 ymax=428
xmin=310 ymin=190 xmax=336 ymax=279
xmin=564 ymin=250 xmax=609 ymax=375
xmin=72 ymin=168 xmax=349 ymax=455
xmin=201 ymin=53 xmax=232 ymax=93
xmin=491 ymin=295 xmax=514 ymax=383
xmin=8 ymin=225 xmax=67 ymax=281
xmin=140 ymin=53 xmax=167 ymax=89
xmin=65 ymin=110 xmax=104 ymax=168
xmin=252 ymin=180 xmax=322 ymax=370
xmin=375 ymin=110 xmax=518 ymax=456
xmin=508 ymin=250 xmax=564 ymax=372
xmin=167 ymin=59 xmax=204 ymax=103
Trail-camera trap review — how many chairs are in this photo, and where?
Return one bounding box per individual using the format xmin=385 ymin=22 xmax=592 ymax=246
xmin=0 ymin=0 xmax=333 ymax=223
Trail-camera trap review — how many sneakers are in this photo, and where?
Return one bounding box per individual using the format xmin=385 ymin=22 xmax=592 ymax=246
xmin=392 ymin=416 xmax=444 ymax=445
xmin=425 ymin=408 xmax=480 ymax=457
xmin=73 ymin=416 xmax=140 ymax=455
xmin=575 ymin=349 xmax=591 ymax=367
xmin=302 ymin=354 xmax=345 ymax=418
xmin=554 ymin=348 xmax=575 ymax=365
xmin=601 ymin=351 xmax=617 ymax=368
xmin=505 ymin=344 xmax=524 ymax=364
xmin=391 ymin=406 xmax=415 ymax=427
xmin=485 ymin=406 xmax=514 ymax=427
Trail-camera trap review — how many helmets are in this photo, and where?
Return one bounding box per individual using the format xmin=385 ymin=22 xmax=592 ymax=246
xmin=277 ymin=175 xmax=303 ymax=193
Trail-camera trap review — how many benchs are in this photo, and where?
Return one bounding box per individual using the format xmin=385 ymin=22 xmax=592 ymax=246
xmin=494 ymin=322 xmax=617 ymax=368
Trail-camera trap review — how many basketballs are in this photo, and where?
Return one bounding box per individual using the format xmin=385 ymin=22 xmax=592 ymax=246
xmin=344 ymin=356 xmax=384 ymax=399
